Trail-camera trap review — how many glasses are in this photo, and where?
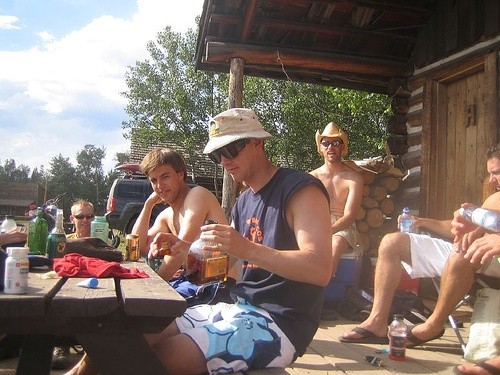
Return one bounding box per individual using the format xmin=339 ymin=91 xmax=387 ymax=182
xmin=73 ymin=215 xmax=93 ymax=219
xmin=207 ymin=138 xmax=250 ymax=164
xmin=320 ymin=139 xmax=343 ymax=148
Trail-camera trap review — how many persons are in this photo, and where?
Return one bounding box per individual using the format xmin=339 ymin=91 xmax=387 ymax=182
xmin=29 ymin=203 xmax=37 ymax=222
xmin=76 ymin=109 xmax=332 ymax=375
xmin=52 ymin=201 xmax=112 ymax=370
xmin=339 ymin=142 xmax=500 ymax=348
xmin=64 ymin=148 xmax=227 ymax=375
xmin=309 ymin=122 xmax=363 ymax=281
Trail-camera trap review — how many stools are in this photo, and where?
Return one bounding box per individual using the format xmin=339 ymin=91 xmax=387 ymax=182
xmin=430 ymin=275 xmax=499 ymax=358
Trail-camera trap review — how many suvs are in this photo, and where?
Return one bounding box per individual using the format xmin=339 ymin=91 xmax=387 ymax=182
xmin=106 ymin=173 xmax=222 ymax=236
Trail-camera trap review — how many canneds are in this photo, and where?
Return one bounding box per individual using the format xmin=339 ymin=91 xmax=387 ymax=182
xmin=46 ymin=233 xmax=67 ymax=258
xmin=124 ymin=234 xmax=141 ymax=262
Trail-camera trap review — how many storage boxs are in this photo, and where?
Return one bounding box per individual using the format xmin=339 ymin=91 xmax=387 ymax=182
xmin=332 ymin=249 xmax=364 ymax=285
xmin=369 ymin=258 xmax=420 ymax=294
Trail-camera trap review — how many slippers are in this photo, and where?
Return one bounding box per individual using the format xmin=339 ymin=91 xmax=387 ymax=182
xmin=339 ymin=326 xmax=389 ymax=344
xmin=406 ymin=324 xmax=445 ymax=348
xmin=454 ymin=361 xmax=500 ymax=375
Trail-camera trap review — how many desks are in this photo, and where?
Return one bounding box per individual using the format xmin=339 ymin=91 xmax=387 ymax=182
xmin=1 ymin=260 xmax=186 ymax=375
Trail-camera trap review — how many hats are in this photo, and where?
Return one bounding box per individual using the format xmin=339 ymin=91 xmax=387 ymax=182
xmin=315 ymin=122 xmax=348 ymax=157
xmin=202 ymin=108 xmax=272 ymax=154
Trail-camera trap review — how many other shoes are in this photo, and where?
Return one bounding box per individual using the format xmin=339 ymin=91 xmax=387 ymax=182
xmin=51 ymin=345 xmax=70 ymax=369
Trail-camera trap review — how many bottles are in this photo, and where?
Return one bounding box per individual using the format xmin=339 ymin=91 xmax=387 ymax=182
xmin=187 ymin=220 xmax=228 ymax=285
xmin=400 ymin=207 xmax=413 ymax=232
xmin=388 ymin=313 xmax=408 ymax=360
xmin=459 ymin=205 xmax=500 ymax=232
xmin=3 ymin=246 xmax=30 ymax=294
xmin=45 ymin=208 xmax=67 ymax=271
xmin=90 ymin=216 xmax=109 ymax=243
xmin=29 ymin=207 xmax=48 ymax=254
xmin=1 ymin=215 xmax=17 ymax=233
